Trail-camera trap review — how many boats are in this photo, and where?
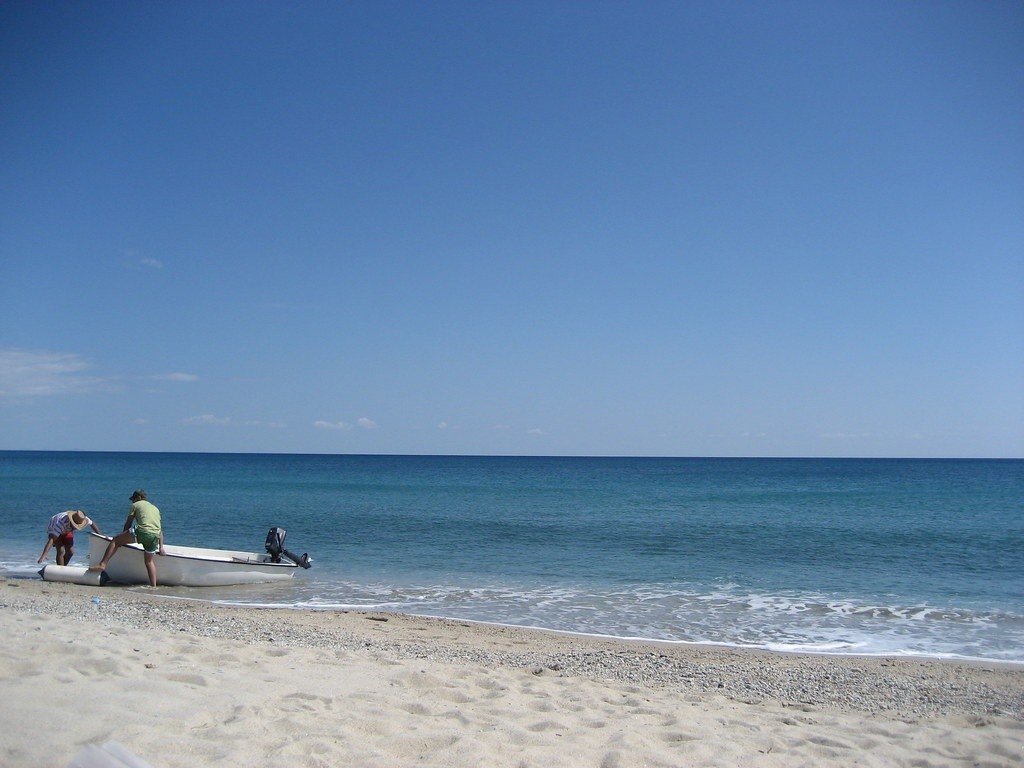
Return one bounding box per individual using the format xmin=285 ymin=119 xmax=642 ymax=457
xmin=87 ymin=527 xmax=315 ymax=587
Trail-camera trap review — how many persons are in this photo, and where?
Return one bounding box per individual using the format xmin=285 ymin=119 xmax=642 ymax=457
xmin=36 ymin=509 xmax=102 ymax=566
xmin=88 ymin=490 xmax=164 ymax=588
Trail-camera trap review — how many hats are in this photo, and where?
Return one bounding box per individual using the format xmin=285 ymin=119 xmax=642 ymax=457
xmin=68 ymin=510 xmax=88 ymax=530
xmin=129 ymin=489 xmax=146 ymax=501
